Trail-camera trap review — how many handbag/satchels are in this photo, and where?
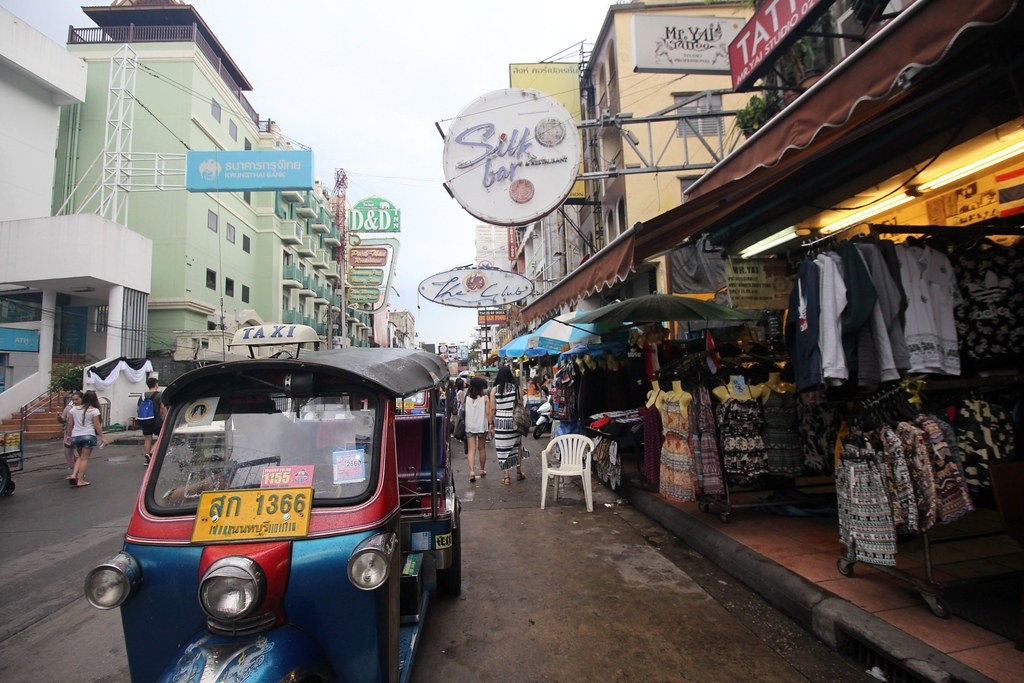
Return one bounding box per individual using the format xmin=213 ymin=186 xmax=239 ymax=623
xmin=513 ymin=385 xmax=529 ymax=437
xmin=454 ymin=394 xmax=466 ymax=440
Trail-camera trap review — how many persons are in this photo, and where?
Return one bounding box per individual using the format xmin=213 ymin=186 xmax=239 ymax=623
xmin=57 ymin=390 xmax=108 ymax=485
xmin=524 ymin=374 xmax=551 ymax=397
xmin=161 ymin=394 xmax=314 ymax=508
xmin=460 ymin=377 xmax=490 ymax=483
xmin=138 ymin=378 xmax=168 ymax=466
xmin=643 ymin=372 xmax=806 ymax=502
xmin=488 ymin=367 xmax=525 ymax=485
xmin=448 ymin=378 xmax=465 ymax=443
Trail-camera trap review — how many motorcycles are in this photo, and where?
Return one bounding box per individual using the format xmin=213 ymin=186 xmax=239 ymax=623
xmin=533 ymin=384 xmax=555 ymax=439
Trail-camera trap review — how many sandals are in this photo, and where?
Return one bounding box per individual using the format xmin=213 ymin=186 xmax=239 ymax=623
xmin=479 ymin=470 xmax=487 ymax=478
xmin=76 ymin=479 xmax=92 ymax=486
xmin=67 ymin=475 xmax=78 ymax=486
xmin=500 ymin=476 xmax=510 ymax=485
xmin=515 ymin=472 xmax=526 ymax=480
xmin=469 ymin=472 xmax=476 ymax=482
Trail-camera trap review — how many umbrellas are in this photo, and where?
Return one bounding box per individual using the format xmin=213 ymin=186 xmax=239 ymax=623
xmin=497 ymin=311 xmax=634 ymax=357
xmin=561 ymin=292 xmax=757 ymax=324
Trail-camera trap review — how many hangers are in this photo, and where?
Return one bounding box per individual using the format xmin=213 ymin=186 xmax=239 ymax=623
xmin=650 ymin=341 xmax=769 ymax=384
xmin=799 ymin=219 xmax=1023 ymax=264
xmin=845 ymin=376 xmax=935 ymax=456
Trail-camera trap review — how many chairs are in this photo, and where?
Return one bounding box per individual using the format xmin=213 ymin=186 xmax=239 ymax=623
xmin=541 ymin=434 xmax=595 ymax=513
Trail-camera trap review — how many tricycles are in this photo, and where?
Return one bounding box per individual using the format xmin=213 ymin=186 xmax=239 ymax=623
xmin=83 ymin=322 xmax=462 ymax=682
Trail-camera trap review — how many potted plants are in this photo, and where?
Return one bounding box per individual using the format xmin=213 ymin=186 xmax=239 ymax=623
xmin=734 ymin=36 xmax=828 ymax=140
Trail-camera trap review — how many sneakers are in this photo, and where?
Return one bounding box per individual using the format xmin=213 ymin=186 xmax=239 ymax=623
xmin=146 ymin=453 xmax=152 ymax=461
xmin=144 ymin=460 xmax=150 ymax=466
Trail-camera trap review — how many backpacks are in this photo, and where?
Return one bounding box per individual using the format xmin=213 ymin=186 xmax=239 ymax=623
xmin=137 ymin=391 xmax=160 ymax=426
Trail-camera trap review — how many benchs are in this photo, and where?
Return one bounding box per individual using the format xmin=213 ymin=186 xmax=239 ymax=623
xmin=292 ymin=413 xmax=447 ymax=508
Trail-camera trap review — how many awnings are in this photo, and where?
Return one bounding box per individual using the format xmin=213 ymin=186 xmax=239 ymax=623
xmin=520 ymin=84 xmax=917 ymax=327
xmin=683 ymin=0 xmax=1024 ymax=203
